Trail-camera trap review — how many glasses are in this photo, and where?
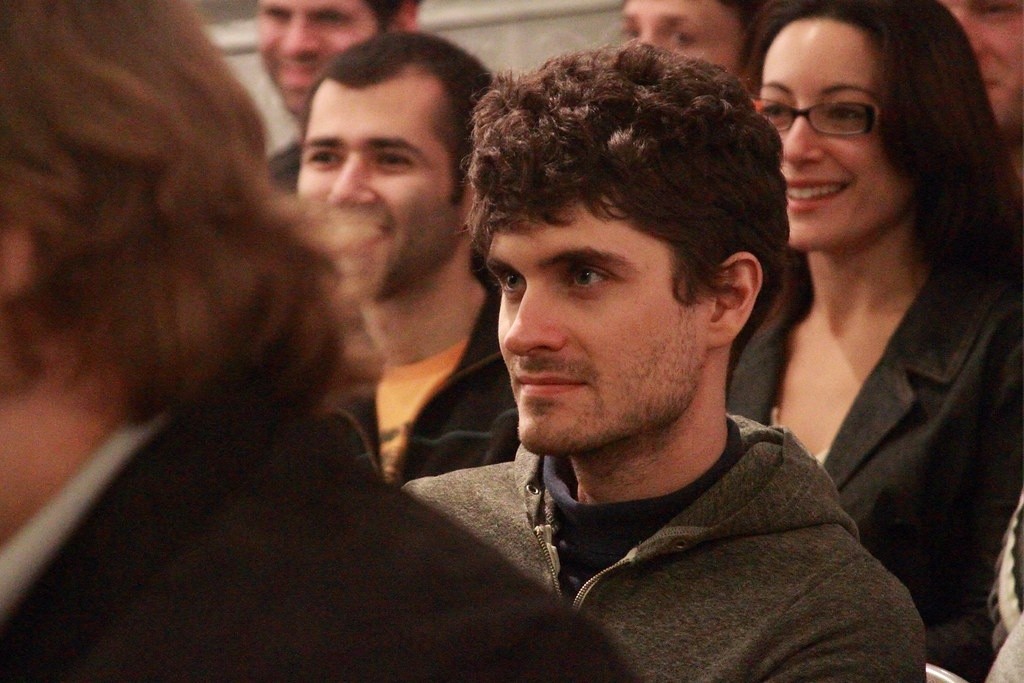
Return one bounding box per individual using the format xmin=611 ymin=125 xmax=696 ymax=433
xmin=749 ymin=98 xmax=874 ymax=136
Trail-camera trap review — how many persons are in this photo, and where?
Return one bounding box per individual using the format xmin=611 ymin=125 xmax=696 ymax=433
xmin=296 ymin=30 xmax=522 ymax=486
xmin=258 ymin=1 xmax=419 ymax=193
xmin=1 ymin=1 xmax=636 ymax=683
xmin=397 ymin=45 xmax=928 ymax=683
xmin=616 ymin=1 xmax=1024 ymax=681
